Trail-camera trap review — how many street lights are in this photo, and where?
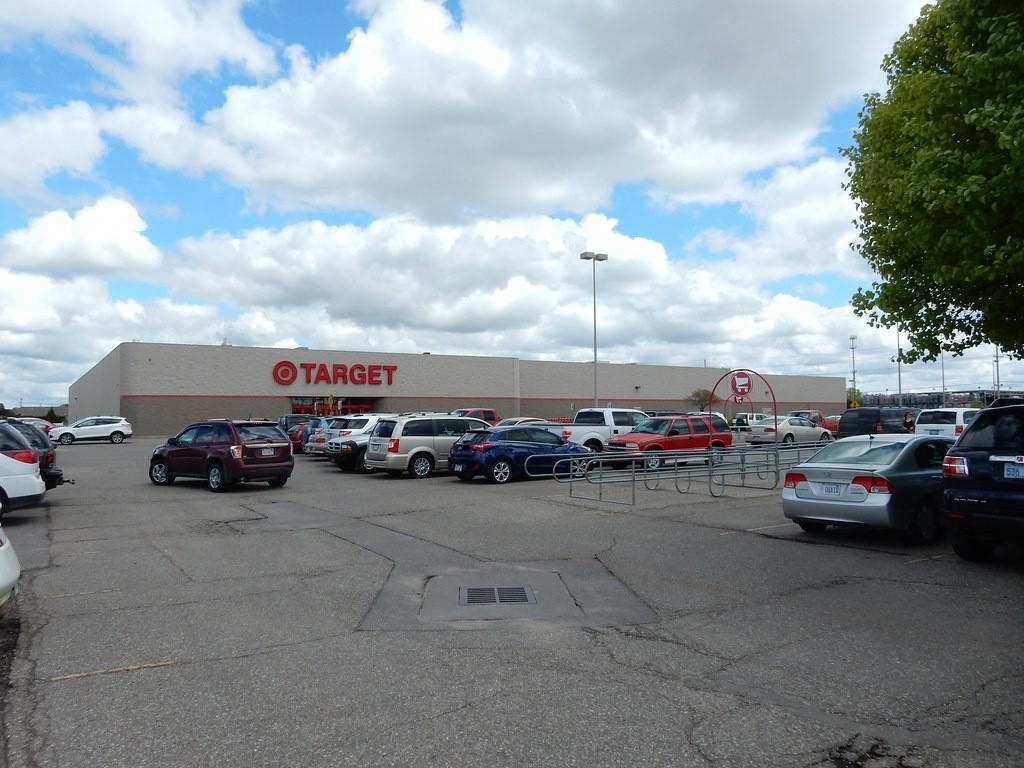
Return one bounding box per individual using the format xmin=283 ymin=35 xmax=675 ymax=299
xmin=580 ymin=251 xmax=609 ymax=406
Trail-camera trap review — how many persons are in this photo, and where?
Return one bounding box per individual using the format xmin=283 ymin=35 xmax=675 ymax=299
xmin=901 ymin=411 xmax=915 ymax=434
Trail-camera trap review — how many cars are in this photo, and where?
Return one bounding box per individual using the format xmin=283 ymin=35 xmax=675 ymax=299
xmin=744 ymin=415 xmax=832 ymax=449
xmin=0 ymin=504 xmax=21 ymax=609
xmin=494 ymin=417 xmax=548 ymax=426
xmin=447 ymin=425 xmax=594 ymax=484
xmin=278 ymin=414 xmax=400 ymax=473
xmin=782 ymin=434 xmax=956 ymax=545
xmin=826 ymin=415 xmax=841 ymax=420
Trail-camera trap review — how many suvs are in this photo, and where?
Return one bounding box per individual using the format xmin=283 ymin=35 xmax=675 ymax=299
xmin=48 ymin=415 xmax=133 ymax=445
xmin=149 ymin=418 xmax=294 ymax=493
xmin=0 ymin=417 xmax=76 ymax=492
xmin=990 ymin=397 xmax=1024 ymax=408
xmin=941 ymin=404 xmax=1024 ymax=562
xmin=837 ymin=406 xmax=920 ymax=439
xmin=604 ymin=413 xmax=734 ymax=471
xmin=0 ymin=419 xmax=46 ymax=517
xmin=732 ymin=413 xmax=767 ymax=431
xmin=15 ymin=417 xmax=54 ymax=434
xmin=449 ymin=408 xmax=500 ymax=426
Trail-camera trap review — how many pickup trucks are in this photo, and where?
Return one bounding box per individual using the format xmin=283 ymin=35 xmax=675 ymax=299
xmin=788 ymin=410 xmax=840 ymax=435
xmin=528 ymin=408 xmax=652 ymax=466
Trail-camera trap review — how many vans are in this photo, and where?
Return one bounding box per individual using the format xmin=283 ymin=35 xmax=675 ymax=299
xmin=914 ymin=408 xmax=981 ymax=439
xmin=364 ymin=412 xmax=493 ymax=478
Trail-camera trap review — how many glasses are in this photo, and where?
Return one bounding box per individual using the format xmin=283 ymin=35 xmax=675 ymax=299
xmin=909 ymin=414 xmax=912 ymax=415
